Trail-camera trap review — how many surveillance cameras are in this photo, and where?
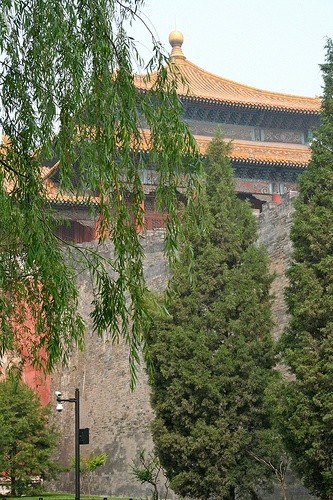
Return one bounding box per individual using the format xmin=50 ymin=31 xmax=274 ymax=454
xmin=54 ymin=390 xmax=63 ymax=398
xmin=56 ymin=403 xmax=63 ymax=413
